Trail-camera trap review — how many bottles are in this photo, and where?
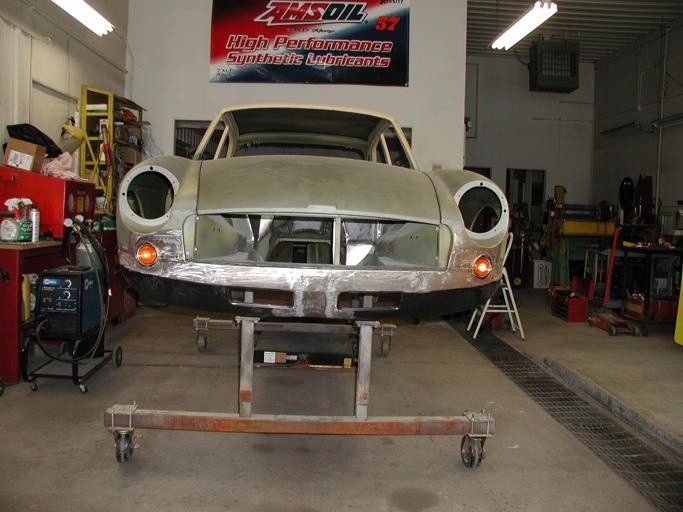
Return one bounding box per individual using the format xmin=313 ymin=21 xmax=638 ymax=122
xmin=29 ymin=204 xmax=41 ymax=241
xmin=0 ymin=212 xmax=22 ymax=242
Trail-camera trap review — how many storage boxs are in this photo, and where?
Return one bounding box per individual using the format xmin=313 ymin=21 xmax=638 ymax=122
xmin=3 ymin=137 xmax=48 ymax=173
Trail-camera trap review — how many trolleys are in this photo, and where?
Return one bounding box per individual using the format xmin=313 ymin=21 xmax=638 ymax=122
xmin=25 ymin=215 xmax=122 ymax=395
xmin=588 ymin=218 xmax=640 ymax=337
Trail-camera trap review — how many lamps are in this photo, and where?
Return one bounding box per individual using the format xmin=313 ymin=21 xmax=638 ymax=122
xmin=484 ymin=0 xmax=558 ymax=54
xmin=46 ymin=0 xmax=115 ymax=38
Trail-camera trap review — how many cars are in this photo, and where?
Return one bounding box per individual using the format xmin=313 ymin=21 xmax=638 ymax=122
xmin=110 ymin=100 xmax=513 ymax=321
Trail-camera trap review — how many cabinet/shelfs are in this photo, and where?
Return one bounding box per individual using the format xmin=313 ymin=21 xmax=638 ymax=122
xmin=78 ymin=84 xmax=152 ymax=224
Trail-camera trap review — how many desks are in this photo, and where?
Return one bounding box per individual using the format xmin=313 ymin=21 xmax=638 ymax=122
xmin=618 ymin=245 xmax=683 ymax=338
xmin=1 ymin=242 xmax=68 ymax=385
